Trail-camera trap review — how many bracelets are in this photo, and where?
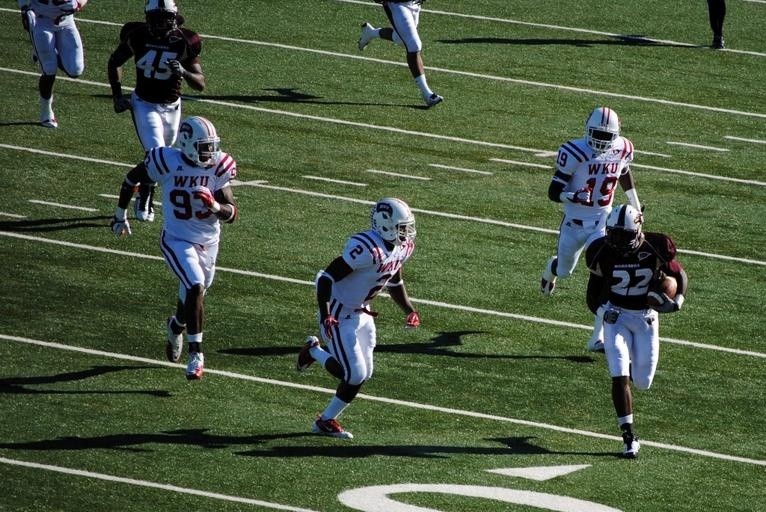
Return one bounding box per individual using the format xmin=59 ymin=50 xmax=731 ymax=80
xmin=596 ymin=306 xmax=607 ymax=321
xmin=673 ymin=294 xmax=687 ymax=311
xmin=209 ymin=200 xmax=222 ymax=214
xmin=115 ymin=207 xmax=127 ymax=221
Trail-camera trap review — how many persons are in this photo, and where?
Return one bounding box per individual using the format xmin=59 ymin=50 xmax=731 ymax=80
xmin=18 ymin=0 xmax=89 ymax=128
xmin=359 ymin=0 xmax=444 ymax=106
xmin=586 ymin=204 xmax=688 ymax=456
xmin=297 ymin=197 xmax=419 ymax=437
xmin=541 ymin=106 xmax=645 ymax=352
xmin=706 ymin=0 xmax=726 ymax=47
xmin=110 ymin=115 xmax=238 ymax=380
xmin=109 ymin=0 xmax=204 ymax=236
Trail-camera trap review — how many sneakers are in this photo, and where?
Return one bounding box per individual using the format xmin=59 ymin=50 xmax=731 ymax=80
xmin=185 ymin=350 xmax=204 ymax=379
xmin=37 ymin=110 xmax=62 ymax=126
xmin=32 ymin=49 xmax=37 ymax=65
xmin=310 ymin=413 xmax=355 ymax=440
xmin=134 ymin=191 xmax=150 ymax=221
xmin=422 ymin=93 xmax=445 ymax=110
xmin=618 ymin=431 xmax=647 ymax=459
xmin=588 ymin=338 xmax=606 ymax=353
xmin=710 ymin=35 xmax=724 ymax=50
xmin=296 ymin=333 xmax=318 ymax=374
xmin=165 ymin=314 xmax=183 ymax=362
xmin=148 ymin=206 xmax=154 ymax=221
xmin=355 ymin=22 xmax=373 ymax=51
xmin=541 ymin=255 xmax=563 ymax=297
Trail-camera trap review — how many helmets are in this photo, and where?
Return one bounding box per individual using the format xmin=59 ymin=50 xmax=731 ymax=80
xmin=370 ymin=196 xmax=417 ymax=246
xmin=175 ymin=117 xmax=222 ymax=172
xmin=603 ymin=203 xmax=645 ymax=254
xmin=583 ymin=105 xmax=623 ymax=150
xmin=143 ymin=1 xmax=178 ymax=40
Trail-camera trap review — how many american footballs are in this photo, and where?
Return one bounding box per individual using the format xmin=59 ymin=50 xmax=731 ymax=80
xmin=654 ymin=274 xmax=679 ymax=303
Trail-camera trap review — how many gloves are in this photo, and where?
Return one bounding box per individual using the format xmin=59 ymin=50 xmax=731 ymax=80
xmin=323 ymin=316 xmax=338 ymax=339
xmin=190 ymin=185 xmax=215 ymax=209
xmin=56 ymin=1 xmax=81 ymax=14
xmin=21 ymin=6 xmax=39 ymax=32
xmin=111 ymin=218 xmax=135 ymax=236
xmin=604 ymin=307 xmax=621 ymax=325
xmin=572 ymin=187 xmax=591 ymax=207
xmin=162 ymin=58 xmax=186 ymax=79
xmin=404 ymin=312 xmax=422 ymax=328
xmin=650 ymin=294 xmax=679 ymax=314
xmin=112 ymin=95 xmax=134 ymax=113
